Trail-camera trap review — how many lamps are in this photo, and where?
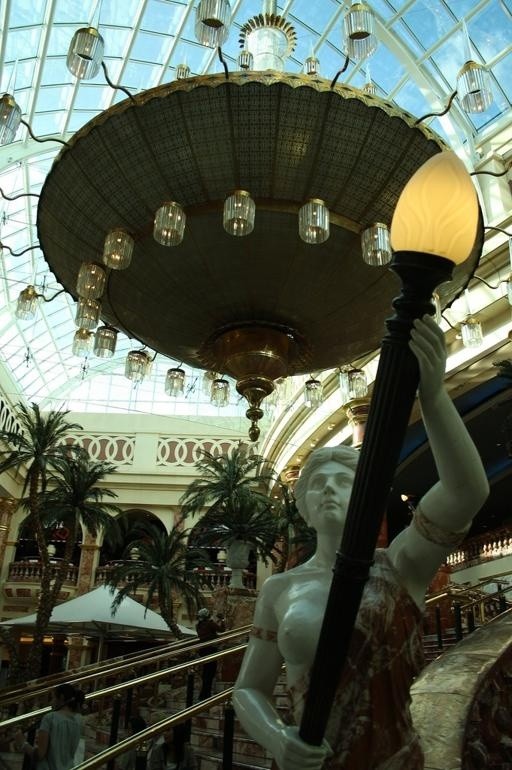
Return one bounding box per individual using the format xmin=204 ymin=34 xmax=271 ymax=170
xmin=0 ymin=0 xmax=512 ymax=445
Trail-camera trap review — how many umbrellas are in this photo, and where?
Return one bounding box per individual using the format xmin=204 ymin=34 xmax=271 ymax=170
xmin=0 ymin=584 xmax=198 ymax=711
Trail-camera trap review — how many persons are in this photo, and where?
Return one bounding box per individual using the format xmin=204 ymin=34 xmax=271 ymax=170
xmin=195 ymin=608 xmax=226 ymax=701
xmin=0 ymin=669 xmax=198 ymax=770
xmin=232 ymin=313 xmax=492 ymax=770
